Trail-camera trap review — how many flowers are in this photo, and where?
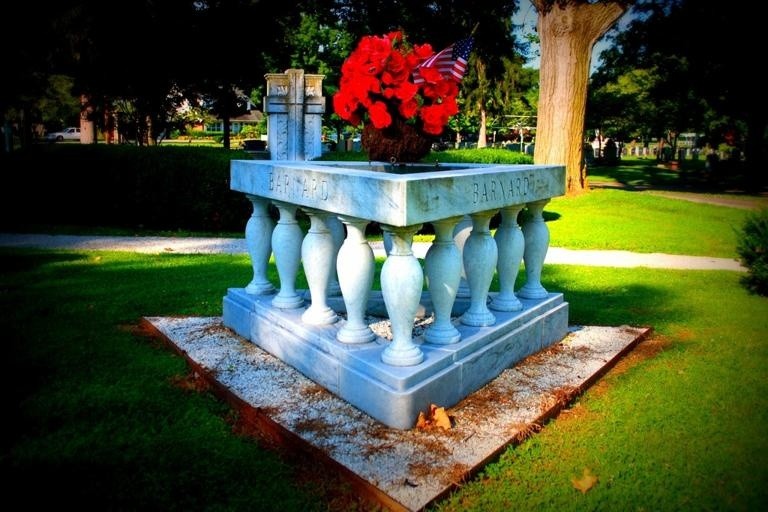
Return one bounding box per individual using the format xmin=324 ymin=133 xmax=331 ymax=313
xmin=332 ymin=31 xmax=461 ymax=135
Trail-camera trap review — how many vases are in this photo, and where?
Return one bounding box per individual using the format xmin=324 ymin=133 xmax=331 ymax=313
xmin=361 ymin=122 xmax=438 ymax=164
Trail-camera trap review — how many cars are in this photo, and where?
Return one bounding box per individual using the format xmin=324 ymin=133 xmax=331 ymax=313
xmin=48 ymin=127 xmax=80 ymax=141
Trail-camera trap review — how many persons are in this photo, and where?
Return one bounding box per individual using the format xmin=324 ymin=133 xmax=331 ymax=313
xmin=586 ymin=134 xmax=705 ymax=162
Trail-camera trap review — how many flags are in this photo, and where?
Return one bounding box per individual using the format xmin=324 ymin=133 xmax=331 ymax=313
xmin=411 ymin=36 xmax=476 ymax=85
